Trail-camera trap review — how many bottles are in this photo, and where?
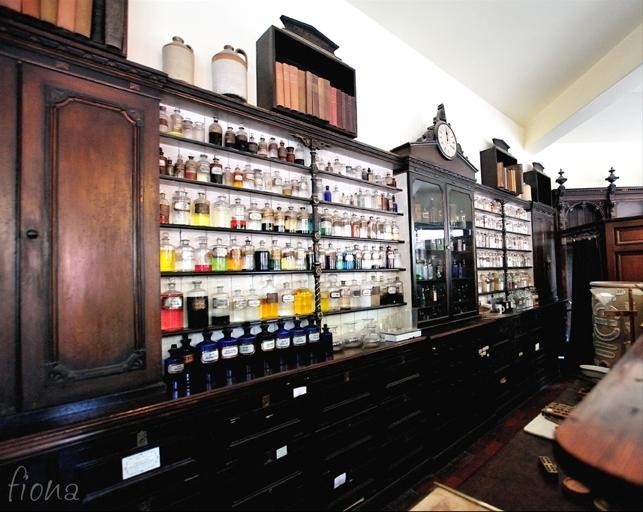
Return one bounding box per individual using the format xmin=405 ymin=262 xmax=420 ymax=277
xmin=161 ymin=104 xmax=539 ymax=390
xmin=162 ymin=36 xmax=194 ymax=85
xmin=211 ymin=45 xmax=248 ymax=104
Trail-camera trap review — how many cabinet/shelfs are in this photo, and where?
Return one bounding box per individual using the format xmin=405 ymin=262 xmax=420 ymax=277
xmin=0 ymin=19 xmax=565 ymax=511
